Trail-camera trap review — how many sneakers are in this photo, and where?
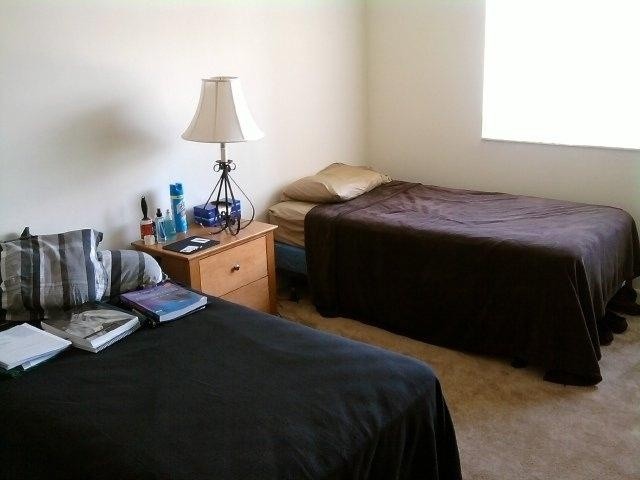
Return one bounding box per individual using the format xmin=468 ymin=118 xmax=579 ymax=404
xmin=604 ymin=310 xmax=628 ymax=333
xmin=614 ymin=285 xmax=637 ymax=302
xmin=597 ymin=321 xmax=614 ymax=345
xmin=607 ymin=296 xmax=640 ymax=316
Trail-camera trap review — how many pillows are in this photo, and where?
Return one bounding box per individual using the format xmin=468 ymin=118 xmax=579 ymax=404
xmin=0 ymin=229 xmax=108 ymax=321
xmin=97 ymin=249 xmax=170 ymax=297
xmin=280 ymin=163 xmax=392 ymax=202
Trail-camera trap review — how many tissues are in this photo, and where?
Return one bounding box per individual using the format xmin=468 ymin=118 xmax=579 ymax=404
xmin=193 ymin=197 xmax=241 ymax=227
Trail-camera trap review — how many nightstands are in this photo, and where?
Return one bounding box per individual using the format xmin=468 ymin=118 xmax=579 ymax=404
xmin=131 ymin=218 xmax=279 ymax=314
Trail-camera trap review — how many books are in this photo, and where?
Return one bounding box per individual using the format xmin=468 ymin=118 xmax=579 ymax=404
xmin=0 ymin=322 xmax=73 ymax=371
xmin=40 ymin=301 xmax=142 ymax=353
xmin=119 ymin=281 xmax=208 ymax=324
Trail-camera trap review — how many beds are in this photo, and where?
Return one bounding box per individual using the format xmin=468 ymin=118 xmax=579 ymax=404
xmin=268 ymin=180 xmax=640 ymax=385
xmin=0 ymin=281 xmax=462 ymax=480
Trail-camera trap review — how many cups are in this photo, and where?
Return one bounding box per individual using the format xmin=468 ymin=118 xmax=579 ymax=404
xmin=144 ymin=234 xmax=155 ymax=246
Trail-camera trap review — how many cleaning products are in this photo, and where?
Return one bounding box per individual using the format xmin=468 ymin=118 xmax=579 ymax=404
xmin=165 ymin=209 xmax=176 ymax=235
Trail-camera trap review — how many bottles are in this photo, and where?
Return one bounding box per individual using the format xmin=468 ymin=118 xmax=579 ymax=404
xmin=164 ymin=209 xmax=176 ymax=236
xmin=170 ymin=182 xmax=187 ymax=233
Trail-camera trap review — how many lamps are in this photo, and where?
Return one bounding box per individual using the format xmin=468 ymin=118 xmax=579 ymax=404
xmin=181 ymin=76 xmax=266 ymax=236
